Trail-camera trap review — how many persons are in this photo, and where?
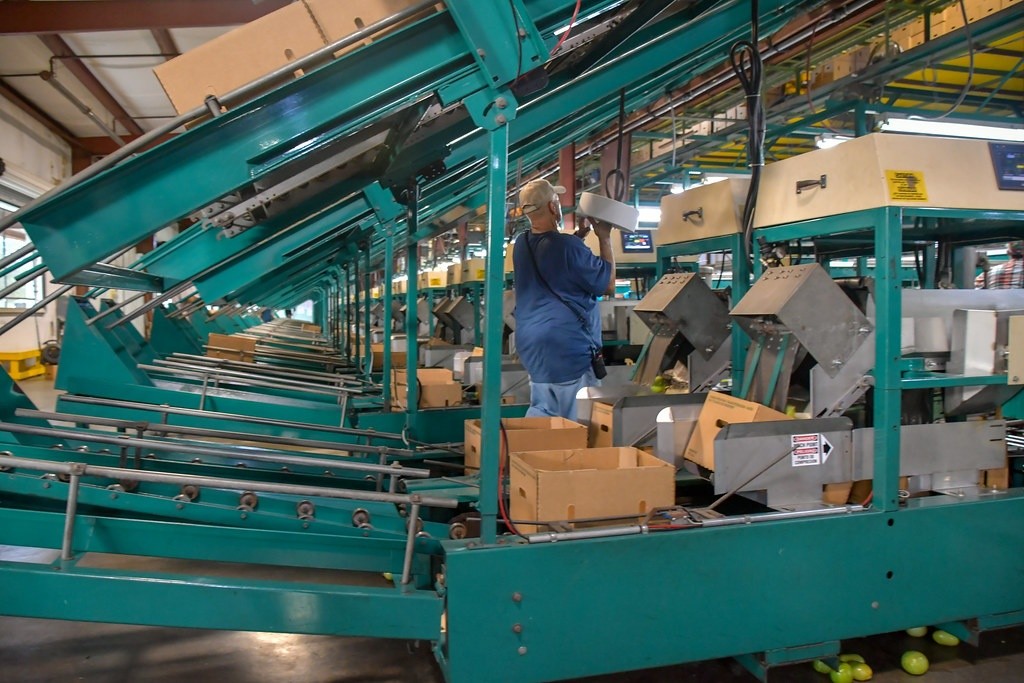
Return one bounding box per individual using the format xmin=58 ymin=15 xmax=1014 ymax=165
xmin=974 ymin=239 xmax=1024 ymax=291
xmin=512 ymin=180 xmax=616 ymax=424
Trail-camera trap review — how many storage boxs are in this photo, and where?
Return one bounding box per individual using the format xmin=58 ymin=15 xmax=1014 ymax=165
xmin=153 ymin=0 xmax=1009 ymax=534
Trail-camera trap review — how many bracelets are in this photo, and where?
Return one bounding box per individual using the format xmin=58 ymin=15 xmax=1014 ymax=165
xmin=573 ymin=231 xmax=585 ymax=241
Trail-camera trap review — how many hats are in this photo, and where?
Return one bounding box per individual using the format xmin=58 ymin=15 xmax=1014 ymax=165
xmin=519 ymin=179 xmax=566 ymax=214
